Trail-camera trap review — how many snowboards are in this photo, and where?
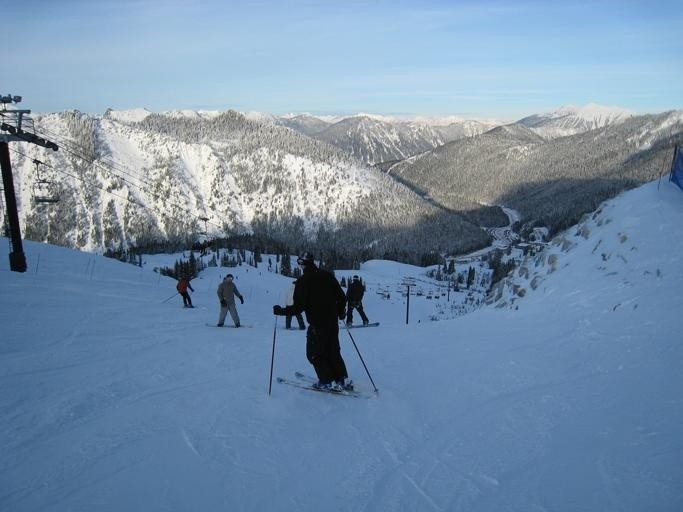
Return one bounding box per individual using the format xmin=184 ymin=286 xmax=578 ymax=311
xmin=339 ymin=323 xmax=379 ymax=330
xmin=279 ymin=326 xmax=307 ymax=330
xmin=206 ymin=323 xmax=252 ymax=328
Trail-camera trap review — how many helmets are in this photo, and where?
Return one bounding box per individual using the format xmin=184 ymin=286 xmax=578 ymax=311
xmin=297 ymin=251 xmax=314 ymax=264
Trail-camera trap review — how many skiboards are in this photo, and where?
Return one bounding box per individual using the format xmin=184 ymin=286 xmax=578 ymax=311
xmin=275 ymin=372 xmax=370 ymax=399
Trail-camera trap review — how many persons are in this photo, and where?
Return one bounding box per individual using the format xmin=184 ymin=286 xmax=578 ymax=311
xmin=217 ymin=274 xmax=244 ymax=327
xmin=274 ymin=249 xmax=352 ymax=392
xmin=345 ymin=274 xmax=369 ymax=325
xmin=285 ymin=281 xmax=306 ymax=330
xmin=176 ymin=274 xmax=195 ymax=308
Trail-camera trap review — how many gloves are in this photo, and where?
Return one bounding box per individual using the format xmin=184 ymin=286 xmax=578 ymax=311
xmin=239 ymin=296 xmax=244 ymax=304
xmin=273 ymin=305 xmax=281 ymax=315
xmin=220 ymin=299 xmax=227 ymax=307
